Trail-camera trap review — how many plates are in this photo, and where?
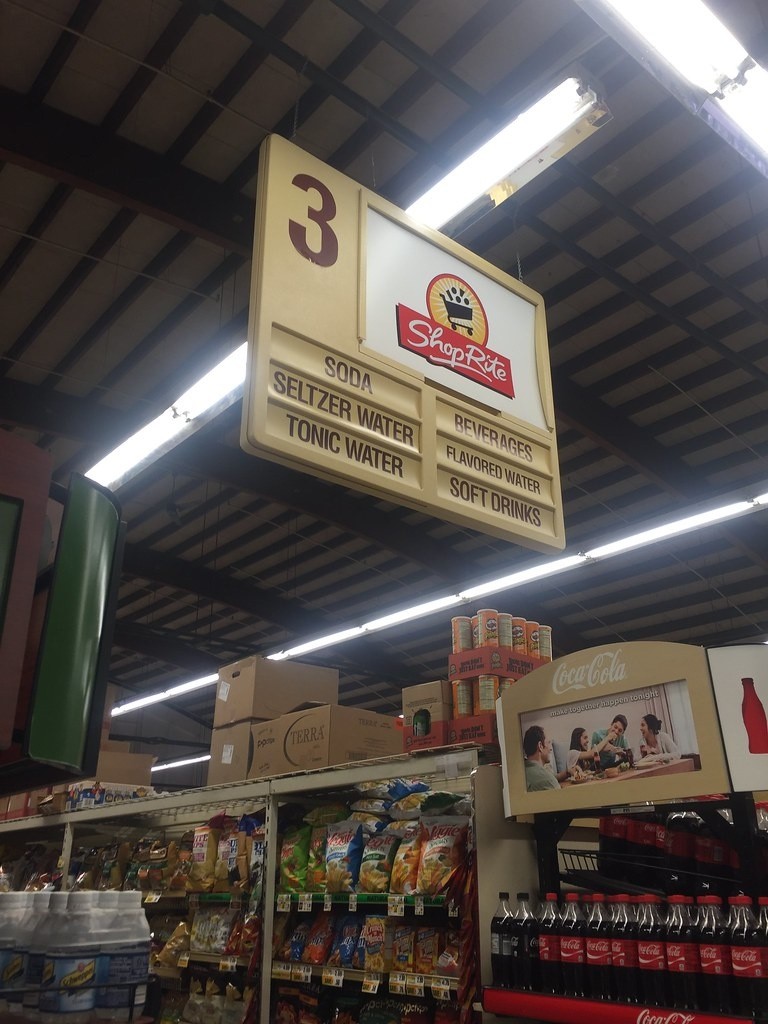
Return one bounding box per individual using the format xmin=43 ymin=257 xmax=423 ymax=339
xmin=568 ymin=777 xmax=586 ymax=784
xmin=631 ymin=762 xmax=658 ymax=768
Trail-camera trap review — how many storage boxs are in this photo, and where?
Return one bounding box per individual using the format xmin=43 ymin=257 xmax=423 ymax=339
xmin=0 ymin=780 xmax=153 ymax=821
xmin=205 ymin=645 xmax=554 ymax=792
xmin=364 ymin=915 xmax=447 ymax=976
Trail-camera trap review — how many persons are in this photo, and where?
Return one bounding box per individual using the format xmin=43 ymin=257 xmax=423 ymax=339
xmin=522 ymin=713 xmax=683 ymax=790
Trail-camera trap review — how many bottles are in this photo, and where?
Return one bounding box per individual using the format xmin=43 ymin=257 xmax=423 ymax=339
xmin=0 ymin=889 xmax=152 ymax=1024
xmin=623 ymin=748 xmax=633 ymax=764
xmin=601 ymin=794 xmax=768 ymax=895
xmin=592 ymin=744 xmax=601 ymax=769
xmin=489 ymin=891 xmax=768 ymax=1024
xmin=739 ymin=677 xmax=768 ymax=754
xmin=640 ymin=744 xmax=647 ymax=759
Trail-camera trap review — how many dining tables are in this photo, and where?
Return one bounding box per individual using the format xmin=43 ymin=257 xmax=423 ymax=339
xmin=558 ymin=757 xmax=695 ymax=786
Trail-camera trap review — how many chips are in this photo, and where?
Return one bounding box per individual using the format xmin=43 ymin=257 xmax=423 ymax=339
xmin=324 ymin=856 xmax=450 ymax=896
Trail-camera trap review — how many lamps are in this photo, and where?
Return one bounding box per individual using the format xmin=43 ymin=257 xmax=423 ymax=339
xmin=50 ymin=0 xmax=768 ymax=774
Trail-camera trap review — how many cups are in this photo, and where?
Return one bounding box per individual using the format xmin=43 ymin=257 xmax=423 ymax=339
xmin=606 ymin=768 xmax=618 ymax=778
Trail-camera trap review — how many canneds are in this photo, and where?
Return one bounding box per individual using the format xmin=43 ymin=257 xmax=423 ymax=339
xmin=413 ymin=710 xmax=428 ymax=737
xmin=433 ymin=609 xmax=552 ymax=722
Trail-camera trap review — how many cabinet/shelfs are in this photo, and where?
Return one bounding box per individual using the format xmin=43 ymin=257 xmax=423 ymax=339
xmin=0 ymin=750 xmax=602 ymax=1024
